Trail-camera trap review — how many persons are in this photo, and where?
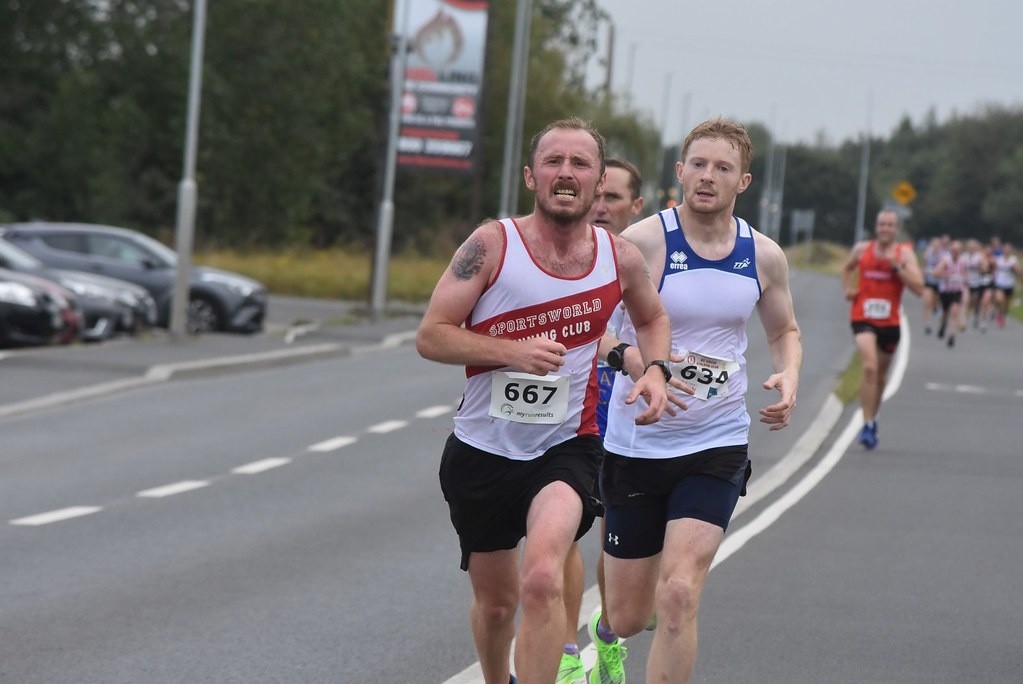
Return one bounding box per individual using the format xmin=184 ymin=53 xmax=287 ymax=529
xmin=557 ymin=158 xmax=644 ymax=684
xmin=843 ymin=207 xmax=926 ymax=446
xmin=598 ymin=119 xmax=803 ymax=684
xmin=923 ymin=234 xmax=1023 ymax=347
xmin=418 ymin=119 xmax=672 ymax=684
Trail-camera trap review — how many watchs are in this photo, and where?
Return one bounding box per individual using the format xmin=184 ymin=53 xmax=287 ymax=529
xmin=893 ymin=263 xmax=902 ymax=272
xmin=644 ymin=360 xmax=671 ymax=383
xmin=607 ymin=343 xmax=632 ymax=376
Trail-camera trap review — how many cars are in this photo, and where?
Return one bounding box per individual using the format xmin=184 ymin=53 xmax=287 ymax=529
xmin=0 ymin=266 xmax=86 ymax=350
xmin=0 ymin=236 xmax=157 ymax=344
xmin=0 ymin=221 xmax=268 ymax=336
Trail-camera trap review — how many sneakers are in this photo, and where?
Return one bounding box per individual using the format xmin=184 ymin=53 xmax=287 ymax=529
xmin=554 ymin=651 xmax=587 ymax=684
xmin=588 ymin=609 xmax=628 ymax=684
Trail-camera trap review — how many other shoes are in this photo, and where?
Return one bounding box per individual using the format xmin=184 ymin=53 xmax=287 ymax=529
xmin=645 ymin=612 xmax=657 ymax=631
xmin=860 ymin=421 xmax=877 ymax=451
xmin=924 ymin=310 xmax=1006 ymax=346
